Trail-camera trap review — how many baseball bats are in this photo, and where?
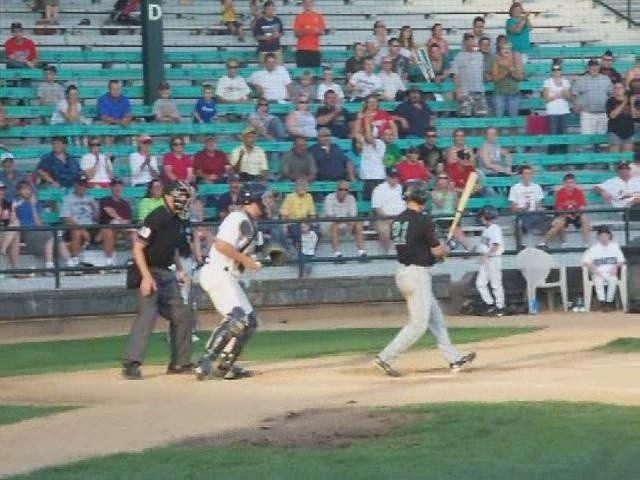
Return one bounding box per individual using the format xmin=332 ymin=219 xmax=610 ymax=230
xmin=447 ymin=171 xmax=479 ymax=245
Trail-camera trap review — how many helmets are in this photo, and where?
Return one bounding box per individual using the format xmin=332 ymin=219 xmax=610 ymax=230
xmin=401 ymin=179 xmax=426 ymax=200
xmin=162 ymin=178 xmax=195 ymax=221
xmin=476 ymin=206 xmax=498 ymax=219
xmin=235 ymin=182 xmax=276 ymax=218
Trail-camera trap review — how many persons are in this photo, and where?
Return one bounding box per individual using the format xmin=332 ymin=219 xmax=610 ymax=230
xmin=476 ymin=202 xmax=507 ymax=316
xmin=508 ymin=50 xmax=640 ymax=250
xmin=34 ymin=12 xmax=90 ymax=35
xmin=190 ymin=182 xmax=280 ymax=379
xmin=370 ymin=179 xmax=478 ymax=377
xmin=40 ymin=65 xmax=217 ymax=126
xmin=213 ymin=16 xmax=524 ymax=266
xmin=121 ymin=180 xmax=199 ymax=380
xmin=6 ymin=22 xmax=38 ymax=68
xmin=580 ymin=225 xmax=629 ymax=313
xmin=0 ymin=135 xmax=230 ymax=277
xmin=115 ymin=0 xmax=140 ymax=25
xmin=34 ymin=1 xmax=58 ymax=23
xmin=221 ymin=0 xmax=327 ymax=67
xmin=505 ymin=2 xmax=534 ymax=62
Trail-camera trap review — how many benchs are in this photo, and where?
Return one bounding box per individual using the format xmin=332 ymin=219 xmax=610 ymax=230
xmin=1 ymin=0 xmax=640 ymax=236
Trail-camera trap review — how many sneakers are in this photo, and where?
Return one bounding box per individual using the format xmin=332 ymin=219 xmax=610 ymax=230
xmin=374 ymin=358 xmax=400 ymax=377
xmin=449 ymin=352 xmax=475 ymax=374
xmin=124 ymin=363 xmax=141 ymax=380
xmin=166 ymin=362 xmax=194 ymax=375
xmin=44 ymin=257 xmax=120 ymax=276
xmin=214 ymin=362 xmax=249 ymax=379
xmin=195 ymin=357 xmax=211 ymax=382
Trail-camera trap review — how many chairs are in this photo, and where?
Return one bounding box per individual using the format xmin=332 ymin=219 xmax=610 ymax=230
xmin=516 ymin=247 xmax=568 ymax=313
xmin=582 ymin=260 xmax=627 ymax=312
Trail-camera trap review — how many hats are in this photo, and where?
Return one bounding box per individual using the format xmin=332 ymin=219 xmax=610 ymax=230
xmin=139 ymin=135 xmax=152 ymax=141
xmin=74 ymin=174 xmax=87 ymax=182
xmin=0 ymin=153 xmax=13 ymax=162
xmin=111 ymin=177 xmax=123 ymax=184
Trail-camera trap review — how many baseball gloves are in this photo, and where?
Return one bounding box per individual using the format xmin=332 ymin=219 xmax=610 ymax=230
xmin=264 ymin=245 xmax=288 ymax=265
